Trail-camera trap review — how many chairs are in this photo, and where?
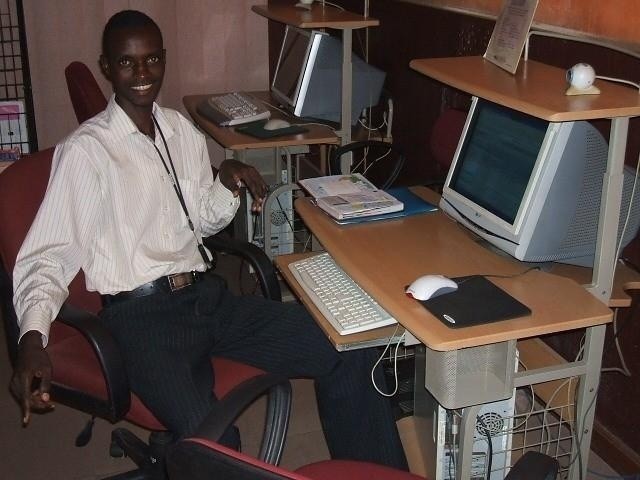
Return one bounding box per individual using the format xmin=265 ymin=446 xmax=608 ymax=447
xmin=65 ymin=61 xmax=107 ymax=123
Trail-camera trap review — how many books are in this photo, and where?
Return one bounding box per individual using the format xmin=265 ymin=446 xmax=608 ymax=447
xmin=310 ymin=186 xmax=439 ymax=225
xmin=298 ymin=167 xmax=405 ymax=219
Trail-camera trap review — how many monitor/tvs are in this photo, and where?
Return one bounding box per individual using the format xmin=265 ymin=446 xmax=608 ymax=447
xmin=439 ymin=96 xmax=639 ymax=263
xmin=270 ymin=25 xmax=385 ymax=127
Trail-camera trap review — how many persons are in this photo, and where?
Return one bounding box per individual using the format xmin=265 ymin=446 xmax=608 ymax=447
xmin=10 ymin=10 xmax=408 ymax=469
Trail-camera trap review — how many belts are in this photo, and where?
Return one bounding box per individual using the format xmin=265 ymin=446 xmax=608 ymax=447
xmin=102 ymin=270 xmax=201 ymax=308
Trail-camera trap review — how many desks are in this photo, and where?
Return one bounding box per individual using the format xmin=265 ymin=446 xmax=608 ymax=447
xmin=184 ymin=4 xmax=379 ymax=255
xmin=292 ymin=52 xmax=637 ymax=480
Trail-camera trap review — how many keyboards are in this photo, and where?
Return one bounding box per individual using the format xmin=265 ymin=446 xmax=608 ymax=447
xmin=197 ymin=90 xmax=271 ymax=128
xmin=288 ymin=253 xmax=398 ymax=336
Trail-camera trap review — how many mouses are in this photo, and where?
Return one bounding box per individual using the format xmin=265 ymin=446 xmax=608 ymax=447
xmin=406 ymin=272 xmax=458 ymax=301
xmin=263 ymin=119 xmax=290 ymax=131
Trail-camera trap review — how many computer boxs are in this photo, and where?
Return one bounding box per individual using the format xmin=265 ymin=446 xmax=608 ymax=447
xmin=232 ymin=148 xmax=293 ymax=274
xmin=413 ymin=339 xmax=519 ymax=480
